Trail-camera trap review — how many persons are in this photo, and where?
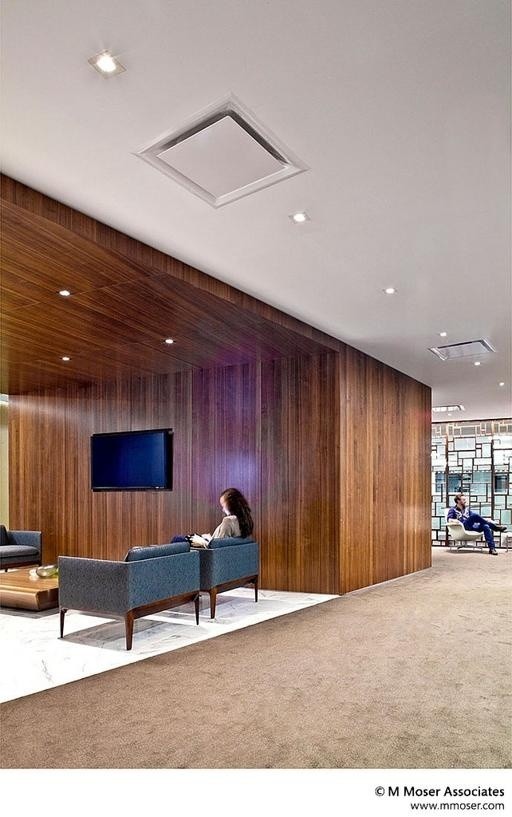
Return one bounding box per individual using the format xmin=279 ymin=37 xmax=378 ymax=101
xmin=169 ymin=486 xmax=253 ymax=548
xmin=447 ymin=494 xmax=507 ymax=555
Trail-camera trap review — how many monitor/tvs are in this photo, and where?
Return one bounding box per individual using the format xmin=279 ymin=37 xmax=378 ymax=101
xmin=89 ymin=428 xmax=173 ymax=490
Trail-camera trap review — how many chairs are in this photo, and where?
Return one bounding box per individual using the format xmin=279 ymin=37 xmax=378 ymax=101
xmin=441 ymin=507 xmax=486 ymax=553
xmin=0 ymin=524 xmax=42 ymax=573
xmin=189 ymin=534 xmax=261 ymax=618
xmin=57 ymin=541 xmax=201 ymax=650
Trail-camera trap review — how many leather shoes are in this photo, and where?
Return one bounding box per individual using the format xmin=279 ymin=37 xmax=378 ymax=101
xmin=498 ymin=527 xmax=507 ymax=531
xmin=489 ymin=547 xmax=498 ymax=555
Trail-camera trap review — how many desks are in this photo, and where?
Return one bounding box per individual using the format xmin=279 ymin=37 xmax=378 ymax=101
xmin=504 ymin=532 xmax=512 ymax=552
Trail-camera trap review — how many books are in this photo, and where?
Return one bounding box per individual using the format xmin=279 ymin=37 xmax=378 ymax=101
xmin=191 ymin=530 xmax=213 ymax=546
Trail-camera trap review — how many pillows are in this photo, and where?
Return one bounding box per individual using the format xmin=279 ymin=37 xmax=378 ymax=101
xmin=124 ymin=543 xmax=158 ymax=562
